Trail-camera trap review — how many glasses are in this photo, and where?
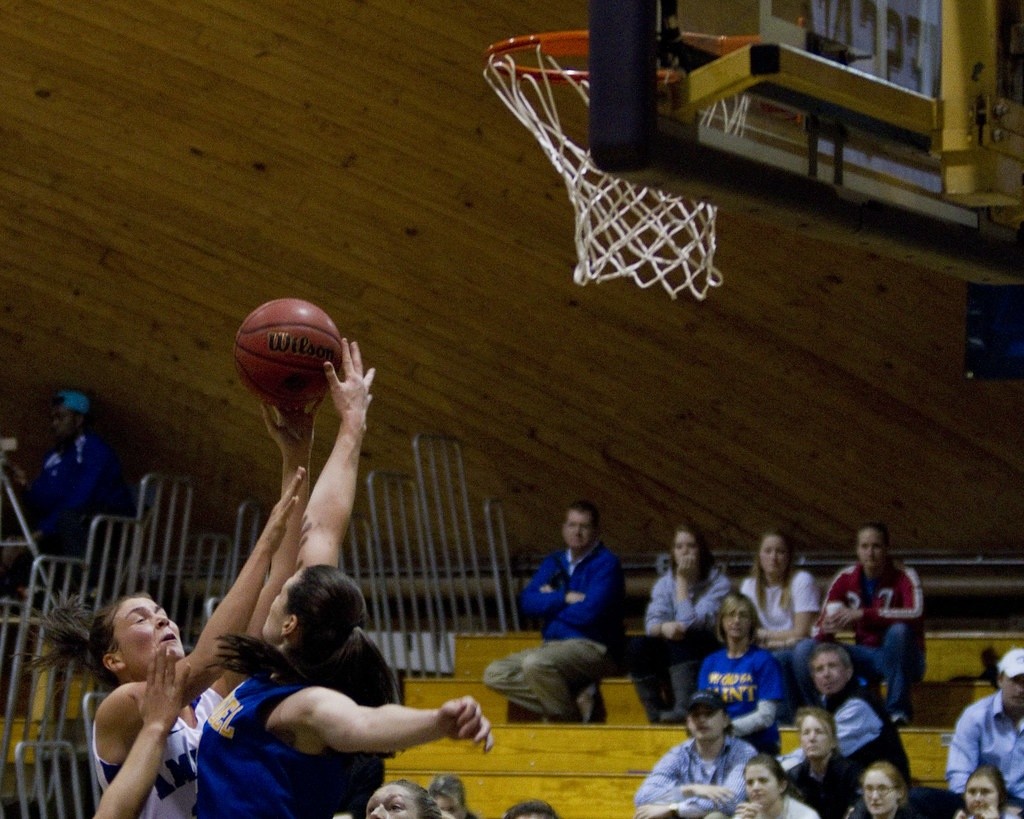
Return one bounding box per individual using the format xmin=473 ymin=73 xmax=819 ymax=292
xmin=51 ymin=413 xmax=72 ymax=421
xmin=864 ymin=784 xmax=899 ymax=796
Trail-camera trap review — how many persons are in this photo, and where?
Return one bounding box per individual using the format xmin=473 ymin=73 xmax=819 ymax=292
xmin=482 ymin=501 xmax=625 ymax=723
xmin=506 ymin=802 xmax=561 ymax=819
xmin=952 ymin=764 xmax=1022 ymax=819
xmin=625 ymin=523 xmax=733 ymax=723
xmin=776 ymin=643 xmax=911 ymax=787
xmin=846 ymin=761 xmax=921 ymax=819
xmin=733 ymin=754 xmax=821 ymax=818
xmin=632 ymin=689 xmax=757 ymax=819
xmin=0 ymin=389 xmax=137 ymax=676
xmin=742 ymin=530 xmax=818 ymax=721
xmin=793 ymin=524 xmax=926 ymax=727
xmin=785 ymin=707 xmax=862 ymax=819
xmin=698 ymin=593 xmax=786 ymax=755
xmin=909 ymin=648 xmax=1024 ymax=819
xmin=1 ymin=339 xmax=494 ymax=819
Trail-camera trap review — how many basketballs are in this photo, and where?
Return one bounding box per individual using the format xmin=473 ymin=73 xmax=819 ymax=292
xmin=233 ymin=298 xmax=344 ymax=409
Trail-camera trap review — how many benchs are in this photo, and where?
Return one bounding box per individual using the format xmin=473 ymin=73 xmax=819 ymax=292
xmin=380 ymin=632 xmax=1024 ymax=819
xmin=0 ymin=642 xmax=106 ymax=763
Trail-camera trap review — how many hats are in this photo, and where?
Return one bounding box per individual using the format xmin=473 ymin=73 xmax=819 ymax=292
xmin=52 ymin=390 xmax=90 ymax=414
xmin=996 ymin=648 xmax=1024 ymax=679
xmin=685 ymin=689 xmax=726 ymax=715
